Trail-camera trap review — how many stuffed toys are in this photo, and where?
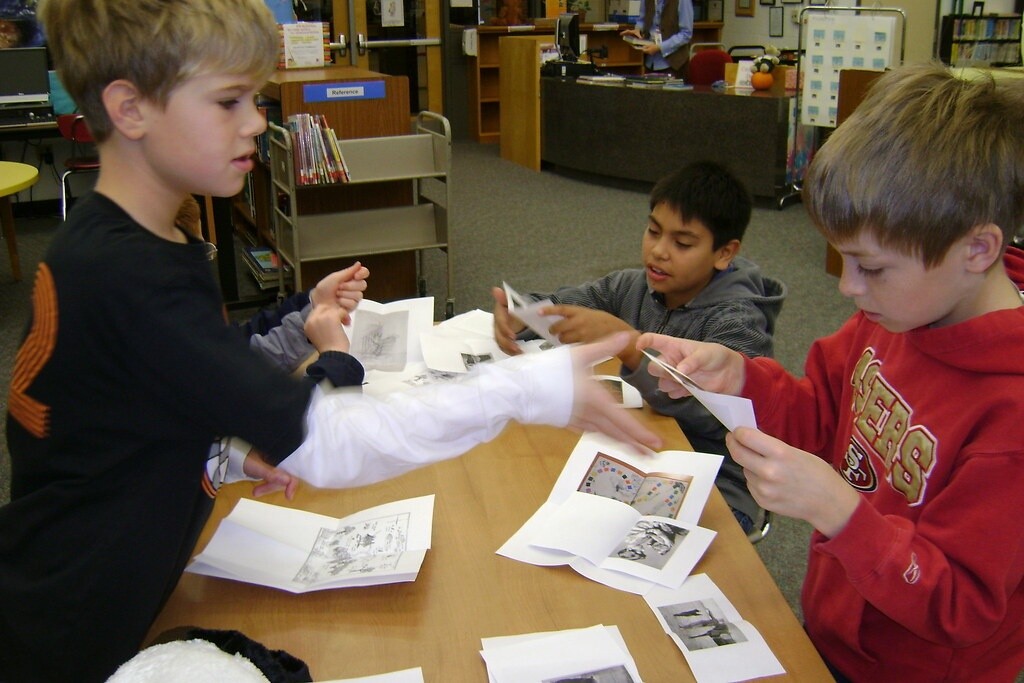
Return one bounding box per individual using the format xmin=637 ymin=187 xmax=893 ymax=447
xmin=750 ymin=44 xmax=781 ymax=73
xmin=488 ymin=0 xmax=526 ymax=25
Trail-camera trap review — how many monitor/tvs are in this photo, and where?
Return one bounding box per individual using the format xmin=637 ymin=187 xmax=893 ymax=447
xmin=553 ymin=13 xmax=581 ymax=62
xmin=0 ymin=46 xmax=51 ymax=103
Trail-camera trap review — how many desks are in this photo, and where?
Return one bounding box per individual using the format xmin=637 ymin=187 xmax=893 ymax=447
xmin=0 ymin=121 xmax=58 ymax=132
xmin=539 ymin=73 xmax=795 ymax=204
xmin=137 ymin=335 xmax=840 ymax=683
xmin=0 ymin=156 xmax=43 ymax=285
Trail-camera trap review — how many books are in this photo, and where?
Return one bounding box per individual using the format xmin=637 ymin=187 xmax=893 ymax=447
xmin=281 ymin=112 xmax=351 ymax=186
xmin=239 ymin=245 xmax=295 ymax=289
xmin=579 ymin=75 xmax=626 ymax=81
xmin=642 ymin=73 xmax=684 ymax=82
xmin=950 ymin=19 xmax=1021 ymax=63
xmin=254 ymin=92 xmax=282 ymax=163
xmin=276 ymin=19 xmax=333 ymax=70
xmin=245 ymin=171 xmax=256 ymax=219
xmin=622 ymin=35 xmax=655 ymax=50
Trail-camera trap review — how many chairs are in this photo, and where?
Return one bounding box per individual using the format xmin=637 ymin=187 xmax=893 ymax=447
xmin=55 ymin=110 xmax=104 ymax=223
xmin=687 ymin=42 xmax=806 ymax=80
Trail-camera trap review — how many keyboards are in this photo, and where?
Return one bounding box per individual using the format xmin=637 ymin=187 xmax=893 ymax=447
xmin=0 ymin=114 xmax=56 ymax=128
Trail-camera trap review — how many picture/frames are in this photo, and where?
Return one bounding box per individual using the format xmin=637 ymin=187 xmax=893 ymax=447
xmin=734 ymin=0 xmax=826 ymax=37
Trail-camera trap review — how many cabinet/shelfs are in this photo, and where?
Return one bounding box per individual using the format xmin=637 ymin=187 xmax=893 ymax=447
xmin=938 ymin=12 xmax=1023 ymax=68
xmin=497 ymin=34 xmax=588 ymax=174
xmin=219 ymin=62 xmax=416 ymax=303
xmin=463 ymin=18 xmax=726 ymax=150
xmin=266 ymin=107 xmax=457 ymax=322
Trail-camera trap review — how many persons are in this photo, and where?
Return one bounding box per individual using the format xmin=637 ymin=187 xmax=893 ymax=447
xmin=634 ymin=64 xmax=1023 ymax=683
xmin=490 ymin=157 xmax=789 ymax=541
xmin=0 ymin=0 xmax=662 ymax=683
xmin=620 ymin=0 xmax=694 ymax=83
xmin=173 ymin=195 xmax=371 ymax=401
